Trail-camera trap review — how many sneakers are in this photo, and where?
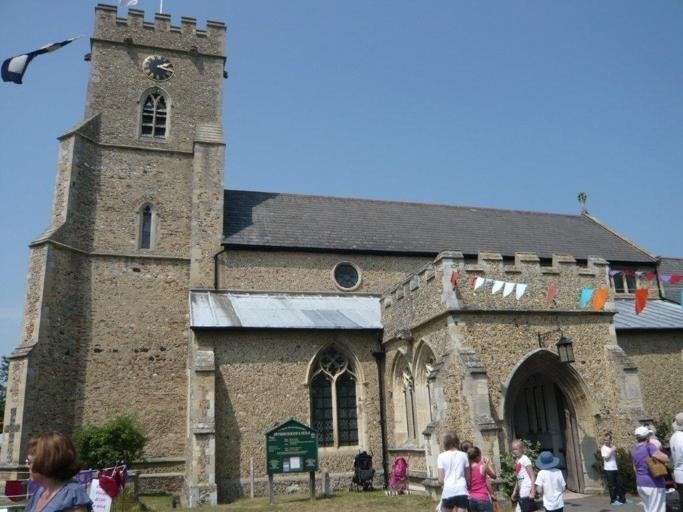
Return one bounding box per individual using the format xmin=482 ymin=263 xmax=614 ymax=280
xmin=611 ymin=499 xmax=633 ymax=505
xmin=666 ymin=488 xmax=676 ymax=493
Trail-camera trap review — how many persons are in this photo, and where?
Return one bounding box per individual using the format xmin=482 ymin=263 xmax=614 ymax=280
xmin=630 ymin=412 xmax=683 ymax=512
xmin=510 ymin=439 xmax=537 ymax=512
xmin=600 ymin=433 xmax=634 ymax=507
xmin=22 ymin=430 xmax=94 ymax=512
xmin=534 ymin=451 xmax=567 ymax=512
xmin=435 ymin=431 xmax=496 ymax=512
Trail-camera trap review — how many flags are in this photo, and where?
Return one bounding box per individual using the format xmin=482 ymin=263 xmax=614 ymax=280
xmin=116 ymin=0 xmax=138 ymax=8
xmin=0 ymin=37 xmax=75 ymax=85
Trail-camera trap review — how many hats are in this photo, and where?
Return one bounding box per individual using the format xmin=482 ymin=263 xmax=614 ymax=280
xmin=633 ymin=427 xmax=655 ymax=438
xmin=535 ymin=452 xmax=560 ymax=470
xmin=671 ymin=413 xmax=682 ymax=431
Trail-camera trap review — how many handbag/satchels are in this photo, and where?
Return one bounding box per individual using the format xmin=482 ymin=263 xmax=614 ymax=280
xmin=646 ymin=457 xmax=668 ymax=477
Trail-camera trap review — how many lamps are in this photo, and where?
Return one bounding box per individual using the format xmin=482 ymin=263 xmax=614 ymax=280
xmin=538 ymin=327 xmax=575 ymax=363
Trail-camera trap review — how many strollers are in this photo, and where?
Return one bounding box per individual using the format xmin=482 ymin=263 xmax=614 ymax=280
xmin=349 ymin=450 xmax=375 ymax=493
xmin=387 ymin=455 xmax=410 ymax=495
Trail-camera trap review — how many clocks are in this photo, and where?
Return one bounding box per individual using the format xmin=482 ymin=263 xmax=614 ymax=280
xmin=142 ymin=55 xmax=174 ymax=82
